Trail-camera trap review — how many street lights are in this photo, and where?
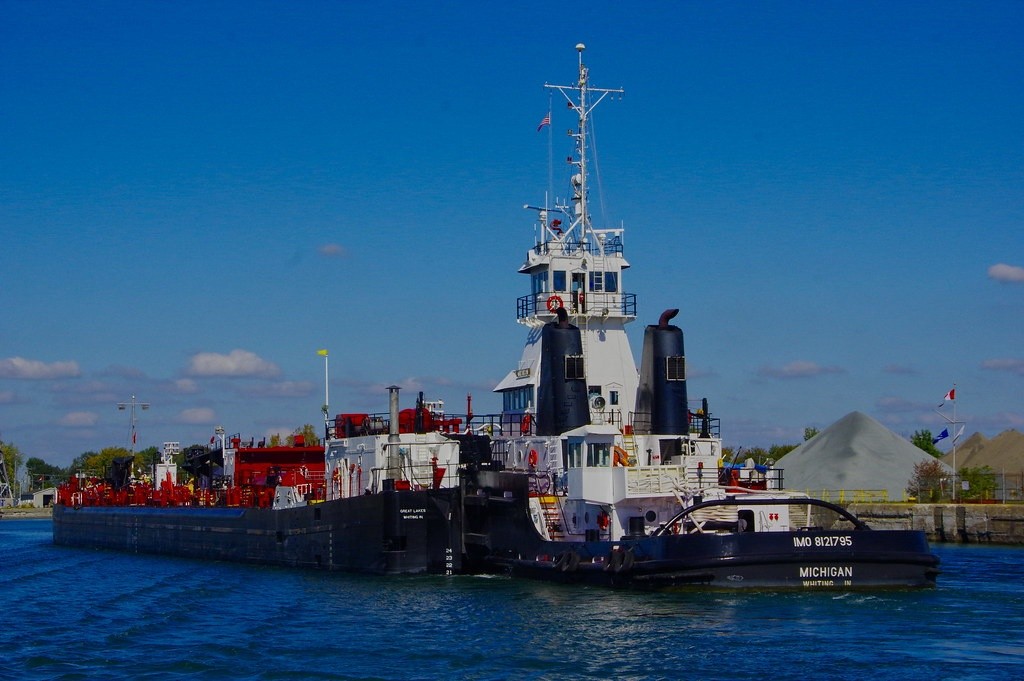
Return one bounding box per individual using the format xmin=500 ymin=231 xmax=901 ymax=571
xmin=315 ymin=348 xmax=331 ymax=438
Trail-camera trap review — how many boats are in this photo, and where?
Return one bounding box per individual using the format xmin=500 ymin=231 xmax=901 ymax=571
xmin=51 ymin=396 xmax=521 ymax=579
xmin=471 ymin=44 xmax=943 ymax=595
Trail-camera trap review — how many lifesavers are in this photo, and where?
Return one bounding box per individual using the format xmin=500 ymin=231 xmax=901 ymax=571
xmin=527 ymin=449 xmax=538 ymax=467
xmin=546 ymin=294 xmax=564 ymax=315
xmin=520 ymin=414 xmax=531 ymax=434
xmin=597 ymin=510 xmax=611 ymax=532
